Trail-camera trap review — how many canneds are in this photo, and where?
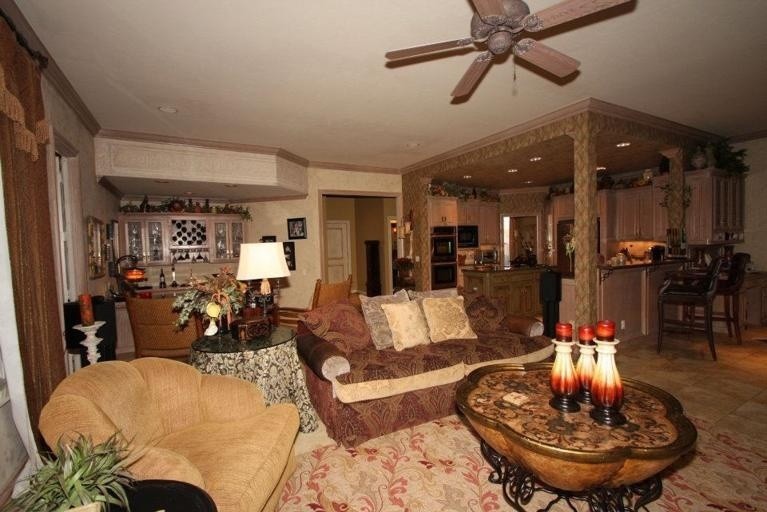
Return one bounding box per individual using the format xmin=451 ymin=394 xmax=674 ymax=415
xmin=616 ymin=252 xmax=625 ymax=266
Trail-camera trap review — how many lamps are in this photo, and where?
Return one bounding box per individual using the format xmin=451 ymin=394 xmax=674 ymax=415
xmin=236 ymin=242 xmax=291 ymax=296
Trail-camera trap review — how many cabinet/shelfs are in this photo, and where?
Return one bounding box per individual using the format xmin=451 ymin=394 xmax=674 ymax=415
xmin=463 ymin=269 xmax=542 ymax=317
xmin=555 ymin=192 xmax=603 ymax=218
xmin=121 ymin=213 xmax=169 ymax=265
xmin=616 ymin=177 xmax=710 ymax=245
xmin=215 ymin=217 xmax=244 ymax=262
xmin=429 ymin=197 xmax=498 ymax=247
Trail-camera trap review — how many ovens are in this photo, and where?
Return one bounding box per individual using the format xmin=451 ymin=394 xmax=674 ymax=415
xmin=430 ymin=228 xmax=458 ymax=289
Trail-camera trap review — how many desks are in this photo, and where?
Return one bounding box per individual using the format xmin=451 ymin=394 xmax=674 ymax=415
xmin=456 ymin=361 xmax=699 ymax=512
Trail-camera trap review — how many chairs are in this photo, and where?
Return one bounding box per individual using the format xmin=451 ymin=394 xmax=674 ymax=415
xmin=657 ymin=253 xmax=750 ymax=360
xmin=279 ymin=274 xmax=353 ymax=325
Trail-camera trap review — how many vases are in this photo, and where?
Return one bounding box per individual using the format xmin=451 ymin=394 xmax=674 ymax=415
xmin=204 ymin=317 xmax=218 ymax=337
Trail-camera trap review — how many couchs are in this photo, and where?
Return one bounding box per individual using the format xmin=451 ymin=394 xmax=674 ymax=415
xmin=296 ymin=287 xmax=555 ymax=449
xmin=39 ymin=358 xmax=299 ymax=512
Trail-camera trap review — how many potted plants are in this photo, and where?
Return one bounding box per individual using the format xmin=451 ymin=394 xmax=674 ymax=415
xmin=3 ymin=428 xmax=149 ymax=512
xmin=657 ymin=181 xmax=695 ymax=248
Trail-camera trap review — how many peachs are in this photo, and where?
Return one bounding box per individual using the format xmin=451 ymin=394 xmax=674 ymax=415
xmin=205 ymin=302 xmax=221 ymax=318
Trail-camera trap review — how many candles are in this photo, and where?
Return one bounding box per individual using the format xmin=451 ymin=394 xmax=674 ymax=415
xmin=554 ymin=318 xmax=619 ymax=343
xmin=79 ymin=292 xmax=95 ymax=325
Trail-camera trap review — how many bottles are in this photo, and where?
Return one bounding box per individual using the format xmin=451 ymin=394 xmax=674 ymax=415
xmin=160 ymin=268 xmax=166 ymax=288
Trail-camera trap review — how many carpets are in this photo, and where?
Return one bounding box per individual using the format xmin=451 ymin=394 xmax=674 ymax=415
xmin=277 ymin=407 xmax=765 ymax=512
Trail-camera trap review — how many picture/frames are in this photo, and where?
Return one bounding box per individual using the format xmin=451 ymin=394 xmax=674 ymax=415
xmin=262 ymin=218 xmax=307 ymax=270
xmin=88 ymin=216 xmax=120 ymax=279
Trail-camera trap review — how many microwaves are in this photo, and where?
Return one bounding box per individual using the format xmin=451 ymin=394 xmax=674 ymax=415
xmin=458 ymin=225 xmax=479 ymax=248
xmin=473 ymin=250 xmax=498 ymax=265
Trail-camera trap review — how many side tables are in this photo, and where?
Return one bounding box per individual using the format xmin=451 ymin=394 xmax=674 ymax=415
xmin=187 ymin=324 xmax=318 ymax=433
xmin=103 ymin=480 xmax=217 ymax=511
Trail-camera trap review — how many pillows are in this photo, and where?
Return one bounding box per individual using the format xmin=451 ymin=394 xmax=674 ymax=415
xmin=295 ymin=285 xmax=509 ymax=356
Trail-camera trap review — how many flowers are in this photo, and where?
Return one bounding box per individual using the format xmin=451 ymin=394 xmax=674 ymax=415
xmin=171 ymin=266 xmax=247 ymax=332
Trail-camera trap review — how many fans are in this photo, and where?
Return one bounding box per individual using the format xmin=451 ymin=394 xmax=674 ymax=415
xmin=385 ymin=1 xmax=638 ymax=98
